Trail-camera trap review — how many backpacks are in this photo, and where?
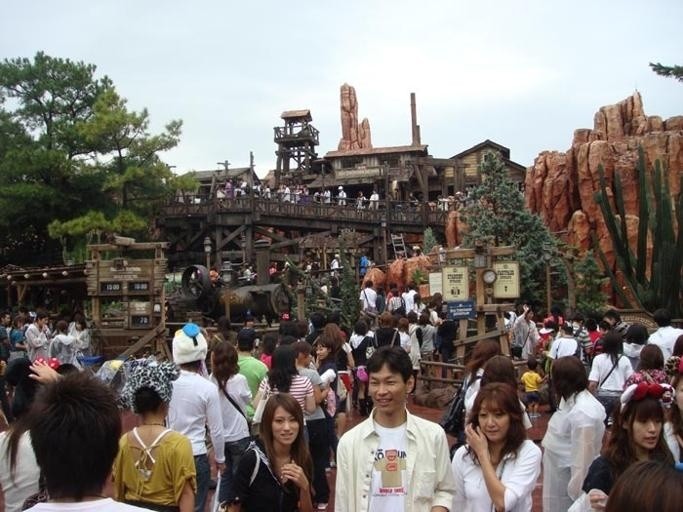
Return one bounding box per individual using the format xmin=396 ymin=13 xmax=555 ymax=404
xmin=438 ymin=374 xmax=482 ymax=438
xmin=511 ymin=347 xmax=521 ymax=358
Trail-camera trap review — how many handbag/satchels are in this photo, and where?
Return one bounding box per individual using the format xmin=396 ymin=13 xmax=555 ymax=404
xmin=366 ymin=306 xmax=378 ymax=318
xmin=251 ymin=377 xmax=269 ymax=425
xmin=207 ymin=469 xmax=229 ymax=512
xmin=335 ymin=376 xmax=347 ymax=402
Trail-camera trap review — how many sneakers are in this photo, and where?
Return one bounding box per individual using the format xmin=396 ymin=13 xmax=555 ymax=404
xmin=317 ymin=503 xmax=328 ymax=510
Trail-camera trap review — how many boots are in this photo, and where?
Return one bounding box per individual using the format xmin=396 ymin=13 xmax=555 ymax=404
xmin=358 ymin=399 xmax=368 ymax=417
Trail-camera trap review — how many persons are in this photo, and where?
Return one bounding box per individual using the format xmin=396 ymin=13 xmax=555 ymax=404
xmin=189 ymin=243 xmax=447 ymax=295
xmin=185 ymin=178 xmax=468 ymax=213
xmin=2 ymin=279 xmax=683 ymax=512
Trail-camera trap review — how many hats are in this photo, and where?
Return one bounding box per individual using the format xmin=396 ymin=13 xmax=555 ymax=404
xmin=237 ymin=327 xmax=258 ymax=344
xmin=118 ymin=353 xmax=179 ymax=417
xmin=538 ymin=327 xmax=554 ymax=335
xmin=171 ymin=323 xmax=207 ymax=366
xmin=597 ymin=335 xmax=612 ymax=345
